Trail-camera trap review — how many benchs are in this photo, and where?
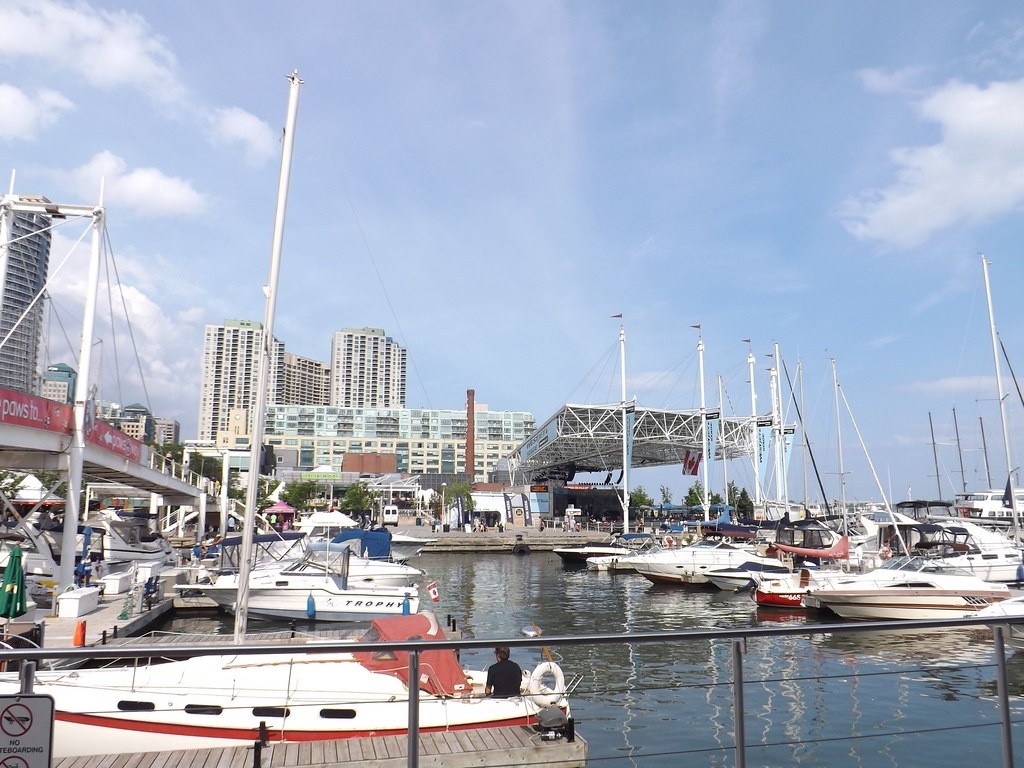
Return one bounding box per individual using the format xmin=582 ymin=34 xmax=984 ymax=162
xmin=57 ymin=587 xmax=101 ymax=617
xmin=199 ymin=559 xmax=218 ymax=568
xmin=137 ymin=560 xmax=162 ymax=583
xmin=101 ymin=571 xmax=134 ymax=595
xmin=157 ymin=568 xmax=185 ymax=594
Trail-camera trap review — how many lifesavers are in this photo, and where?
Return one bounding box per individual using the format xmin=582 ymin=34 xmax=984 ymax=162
xmin=529 ymin=662 xmax=565 ymax=707
xmin=722 ymin=536 xmax=732 ymax=545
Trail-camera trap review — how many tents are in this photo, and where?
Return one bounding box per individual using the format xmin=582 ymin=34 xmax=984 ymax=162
xmin=265 ymin=501 xmax=298 ymax=531
xmin=0 ymin=474 xmax=66 ymax=513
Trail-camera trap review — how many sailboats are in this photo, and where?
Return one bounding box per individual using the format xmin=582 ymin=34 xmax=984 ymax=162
xmin=553 ymin=249 xmax=1024 ymax=654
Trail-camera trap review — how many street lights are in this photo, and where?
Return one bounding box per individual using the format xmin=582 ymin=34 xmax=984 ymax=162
xmin=441 ymin=483 xmax=446 ymax=534
xmin=653 ymin=500 xmax=660 ymax=516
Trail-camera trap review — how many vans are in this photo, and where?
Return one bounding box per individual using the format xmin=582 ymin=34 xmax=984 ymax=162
xmin=383 ymin=505 xmax=399 ymax=527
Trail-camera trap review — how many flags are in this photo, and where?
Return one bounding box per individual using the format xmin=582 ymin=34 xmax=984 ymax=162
xmin=427 ymin=582 xmax=439 ymax=603
xmin=682 ymin=450 xmax=702 ymax=475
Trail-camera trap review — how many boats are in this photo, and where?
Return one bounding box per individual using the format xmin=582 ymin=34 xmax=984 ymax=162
xmin=0 ymin=609 xmax=570 ymax=759
xmin=171 ymin=484 xmax=423 ymax=621
xmin=0 ymin=510 xmax=177 ymax=594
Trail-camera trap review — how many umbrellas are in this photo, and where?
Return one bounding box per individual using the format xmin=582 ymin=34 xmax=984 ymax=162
xmin=0 ymin=544 xmax=27 ymax=640
xmin=293 ymin=511 xmax=358 ymax=583
xmin=77 ymin=525 xmax=94 ymax=588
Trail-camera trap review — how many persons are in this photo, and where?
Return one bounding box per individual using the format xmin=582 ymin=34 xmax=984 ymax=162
xmin=579 ymin=505 xmax=718 ymax=534
xmin=165 ymin=450 xmax=173 ymax=477
xmin=181 ymin=461 xmax=189 ymax=483
xmin=484 ymin=647 xmax=522 ymax=698
xmin=202 ymin=475 xmax=220 ymax=497
xmin=434 ymin=518 xmax=441 ymax=534
xmin=537 ymin=516 xmax=580 ymax=532
xmin=228 ymin=515 xmax=235 ymax=532
xmin=473 ymin=515 xmax=508 ymax=533
xmin=193 ymin=534 xmax=224 ymax=559
